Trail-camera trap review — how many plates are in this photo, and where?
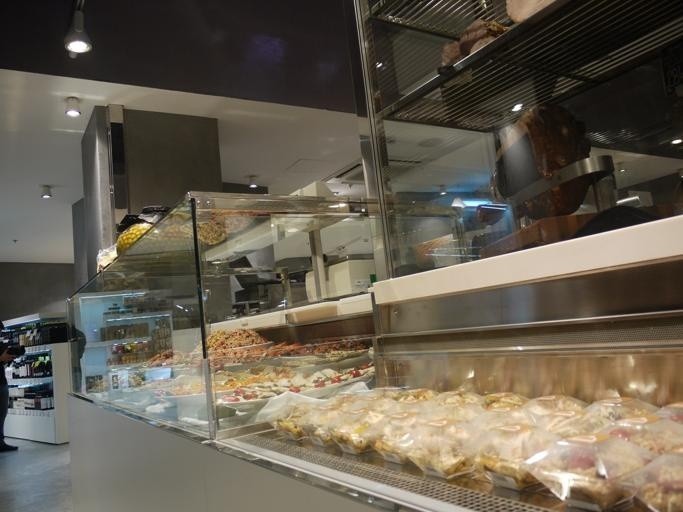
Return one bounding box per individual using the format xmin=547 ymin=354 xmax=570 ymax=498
xmin=219 ymin=400 xmax=268 ymax=411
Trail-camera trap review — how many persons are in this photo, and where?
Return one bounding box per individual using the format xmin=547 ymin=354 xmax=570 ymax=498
xmin=0 ymin=319 xmax=18 ymax=452
xmin=68 ymin=323 xmax=86 ymax=393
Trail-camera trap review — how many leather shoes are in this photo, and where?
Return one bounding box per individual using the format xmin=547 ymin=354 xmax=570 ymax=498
xmin=0 ymin=442 xmax=17 ymax=453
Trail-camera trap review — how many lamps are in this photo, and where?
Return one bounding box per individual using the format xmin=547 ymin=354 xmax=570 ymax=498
xmin=64 ymin=1 xmax=93 ymax=54
xmin=40 ymin=185 xmax=52 ymax=199
xmin=64 ymin=97 xmax=81 ymax=118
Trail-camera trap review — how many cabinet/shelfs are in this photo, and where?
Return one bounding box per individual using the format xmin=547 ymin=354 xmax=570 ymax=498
xmin=73 ymin=288 xmax=176 ymax=396
xmin=2 ymin=312 xmax=72 ymax=445
xmin=351 ymin=1 xmax=683 ymax=308
